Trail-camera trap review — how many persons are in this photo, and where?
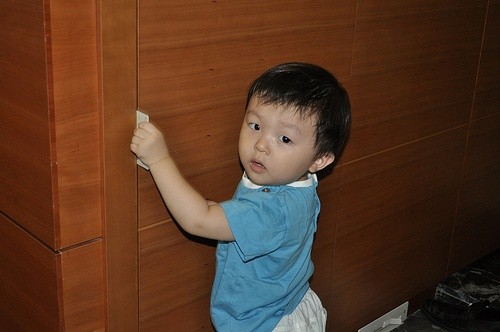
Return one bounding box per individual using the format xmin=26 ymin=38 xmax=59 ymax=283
xmin=130 ymin=61 xmax=352 ymax=331
